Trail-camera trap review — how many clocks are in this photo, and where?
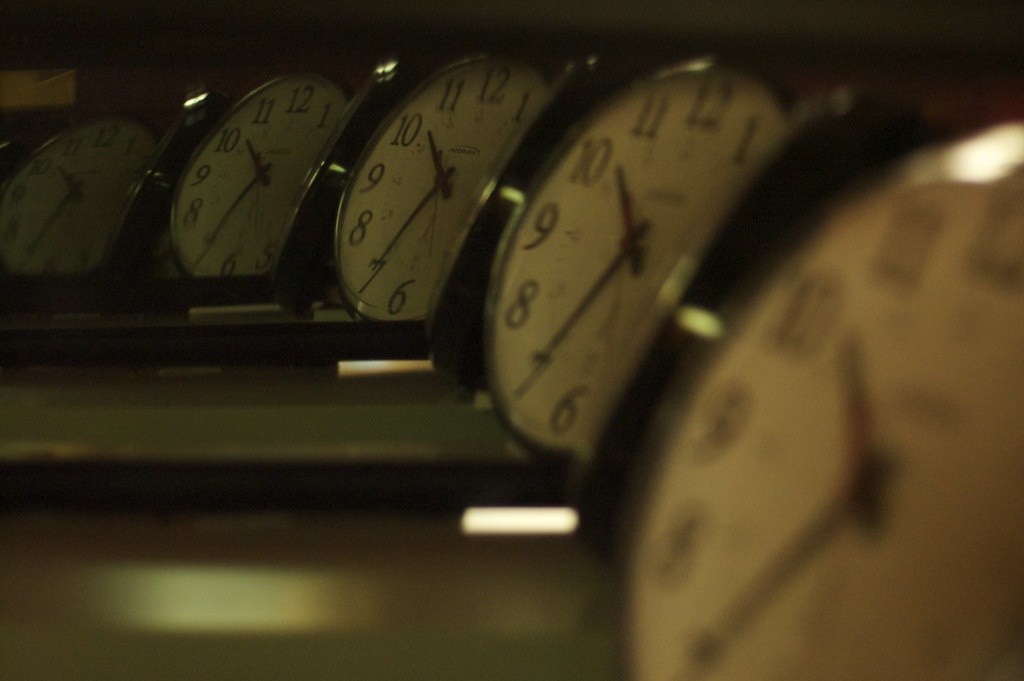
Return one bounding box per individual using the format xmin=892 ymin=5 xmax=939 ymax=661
xmin=0 ymin=111 xmax=160 ymax=283
xmin=433 ymin=45 xmax=799 ymax=478
xmin=330 ymin=46 xmax=569 ymax=327
xmin=168 ymin=67 xmax=357 ymax=280
xmin=617 ymin=97 xmax=1024 ymax=681
